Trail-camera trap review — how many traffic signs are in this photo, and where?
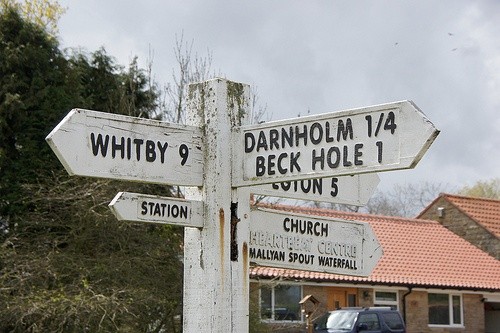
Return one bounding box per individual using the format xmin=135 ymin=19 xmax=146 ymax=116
xmin=43 ymin=74 xmax=442 ymax=333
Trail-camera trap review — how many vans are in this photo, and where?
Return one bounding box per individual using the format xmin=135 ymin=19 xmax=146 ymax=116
xmin=312 ymin=307 xmax=407 ymax=333
